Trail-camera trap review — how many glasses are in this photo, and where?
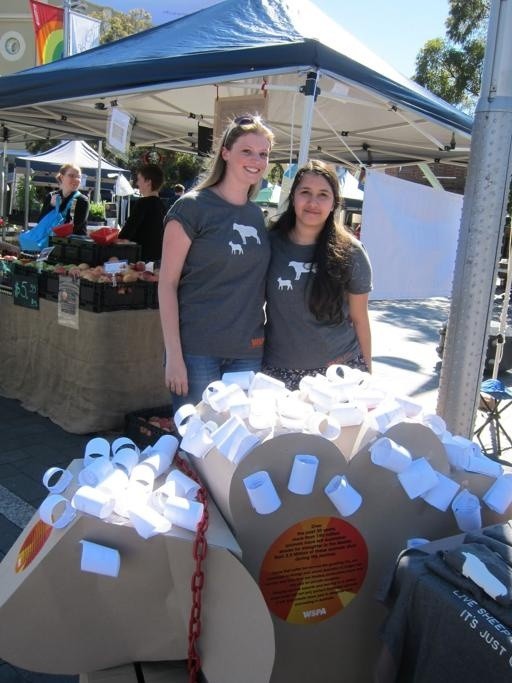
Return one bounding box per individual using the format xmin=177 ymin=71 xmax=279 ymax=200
xmin=223 ymin=115 xmax=273 ymax=148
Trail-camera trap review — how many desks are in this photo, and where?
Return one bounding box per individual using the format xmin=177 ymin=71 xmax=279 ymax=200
xmin=0 ymin=288 xmax=168 ymax=425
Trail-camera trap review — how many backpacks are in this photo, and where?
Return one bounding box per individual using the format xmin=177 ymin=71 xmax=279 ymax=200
xmin=18 ymin=194 xmax=73 ymax=253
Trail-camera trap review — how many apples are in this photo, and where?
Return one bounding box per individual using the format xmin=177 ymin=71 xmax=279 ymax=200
xmin=71 ymin=261 xmax=158 ymax=294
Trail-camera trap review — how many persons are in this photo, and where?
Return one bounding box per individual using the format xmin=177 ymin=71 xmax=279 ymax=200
xmin=168 ymin=183 xmax=184 ymax=214
xmin=118 ymin=164 xmax=166 ymax=268
xmin=264 ymin=159 xmax=375 ymax=391
xmin=38 ymin=164 xmax=90 ymax=236
xmin=158 ymin=114 xmax=270 ymax=421
